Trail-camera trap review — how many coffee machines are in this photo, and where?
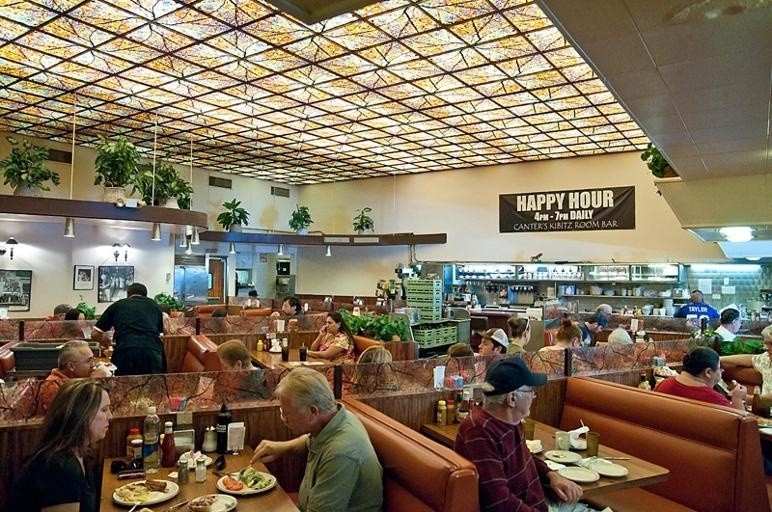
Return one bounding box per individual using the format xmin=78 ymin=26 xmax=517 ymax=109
xmin=509 ymin=284 xmax=536 ymax=305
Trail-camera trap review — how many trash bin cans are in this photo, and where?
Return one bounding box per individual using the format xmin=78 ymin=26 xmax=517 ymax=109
xmin=446 ymin=318 xmax=471 ymax=345
xmin=9 ymin=342 xmax=100 ymax=380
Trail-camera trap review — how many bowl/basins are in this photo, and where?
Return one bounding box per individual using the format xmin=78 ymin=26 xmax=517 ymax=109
xmin=589 ymin=286 xmax=615 ymax=296
xmin=621 ymin=287 xmax=640 ymax=296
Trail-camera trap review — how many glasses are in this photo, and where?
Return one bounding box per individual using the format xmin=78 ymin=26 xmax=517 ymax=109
xmin=278 ymin=406 xmax=297 ymax=426
xmin=518 ymin=387 xmax=537 ymax=397
xmin=110 ymin=458 xmax=144 ymax=474
xmin=717 ymin=368 xmax=724 ymax=373
xmin=521 ymin=317 xmax=530 ymax=335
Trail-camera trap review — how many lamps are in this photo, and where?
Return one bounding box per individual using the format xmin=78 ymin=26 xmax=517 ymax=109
xmin=150 ymin=221 xmax=164 ymax=242
xmin=62 ymin=217 xmax=77 ymax=238
xmin=0 ymin=236 xmax=19 ymax=260
xmin=175 ymin=224 xmax=341 ymax=258
xmin=72 ymin=264 xmax=95 ymax=291
xmin=111 ymin=243 xmax=131 ymax=262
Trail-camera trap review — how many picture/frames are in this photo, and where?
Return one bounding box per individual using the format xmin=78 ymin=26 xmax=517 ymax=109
xmin=0 ymin=268 xmax=34 ymax=312
xmin=96 ymin=264 xmax=136 ymax=304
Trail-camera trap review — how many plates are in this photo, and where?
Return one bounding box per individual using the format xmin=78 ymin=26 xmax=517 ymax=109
xmin=530 ymin=448 xmax=545 ymax=454
xmin=269 ymin=349 xmax=283 ymax=353
xmin=113 ymin=478 xmax=178 ymax=508
xmin=176 ymin=454 xmax=213 ymax=470
xmin=641 ymin=299 xmax=676 ymax=316
xmin=570 ymin=437 xmax=586 ymax=451
xmin=757 ymin=423 xmax=772 ymax=428
xmin=188 ymin=492 xmax=236 ymax=512
xmin=543 ymin=448 xmax=628 ymax=484
xmin=216 ymin=469 xmax=276 ymax=497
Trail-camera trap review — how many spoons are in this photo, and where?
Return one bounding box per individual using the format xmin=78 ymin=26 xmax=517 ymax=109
xmin=239 ymin=464 xmax=254 ymax=479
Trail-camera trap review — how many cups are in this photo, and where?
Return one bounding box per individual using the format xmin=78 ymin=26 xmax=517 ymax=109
xmin=585 ymin=432 xmax=601 ymax=458
xmin=630 ymin=319 xmax=644 ymax=332
xmin=751 ymin=312 xmax=761 ymax=322
xmin=298 ymin=346 xmax=308 ymax=361
xmin=522 ymin=418 xmax=535 ymax=440
xmin=554 ymin=431 xmax=568 ymax=450
xmin=283 ymin=347 xmax=288 ymax=360
xmin=576 ymin=287 xmax=585 ymax=295
xmin=657 ymin=358 xmax=666 ymax=366
xmin=757 ymin=397 xmax=771 ymax=417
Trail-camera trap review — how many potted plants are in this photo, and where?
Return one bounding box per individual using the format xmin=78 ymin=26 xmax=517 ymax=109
xmin=215 ymin=201 xmax=252 ymax=235
xmin=640 ymin=142 xmax=679 ymax=196
xmin=0 ymin=145 xmax=64 ymax=199
xmin=91 ymin=136 xmax=139 ymax=204
xmin=287 ymin=203 xmax=315 ymax=234
xmin=126 ymin=157 xmax=194 ymax=211
xmin=353 ymin=205 xmax=375 ymax=236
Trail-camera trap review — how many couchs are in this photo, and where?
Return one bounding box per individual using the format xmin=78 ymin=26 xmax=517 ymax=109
xmin=1 ymin=296 xmax=771 ymax=511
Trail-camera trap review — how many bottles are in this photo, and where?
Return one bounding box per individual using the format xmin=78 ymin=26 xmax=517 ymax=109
xmin=264 ymin=333 xmax=270 ymax=351
xmin=436 ymin=393 xmax=475 ymax=426
xmin=257 ymin=340 xmax=263 ymax=352
xmin=522 ymin=272 xmax=549 ymax=281
xmin=752 ymin=385 xmax=761 ymax=413
xmin=177 ymin=459 xmax=189 ymax=485
xmin=202 ymin=426 xmax=218 ymax=453
xmin=639 ymin=369 xmax=647 ymax=382
xmin=215 ymin=404 xmax=232 ymax=454
xmin=194 ymin=458 xmax=207 ymax=483
xmin=633 ymin=307 xmax=636 ymax=314
xmin=624 ymin=309 xmax=627 ymax=314
xmin=126 ymin=404 xmax=175 ymax=476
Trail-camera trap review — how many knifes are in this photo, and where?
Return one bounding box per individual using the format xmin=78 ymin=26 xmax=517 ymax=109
xmin=602 ymin=456 xmax=631 ymax=461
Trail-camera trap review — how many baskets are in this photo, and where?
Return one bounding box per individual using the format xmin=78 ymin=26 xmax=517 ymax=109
xmin=405 ymin=280 xmax=442 ymax=323
xmin=412 ymin=325 xmax=458 ymax=350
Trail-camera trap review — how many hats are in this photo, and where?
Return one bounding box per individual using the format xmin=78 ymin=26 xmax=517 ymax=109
xmin=477 ymin=328 xmax=509 ymax=348
xmin=482 ymin=358 xmax=548 ymax=395
